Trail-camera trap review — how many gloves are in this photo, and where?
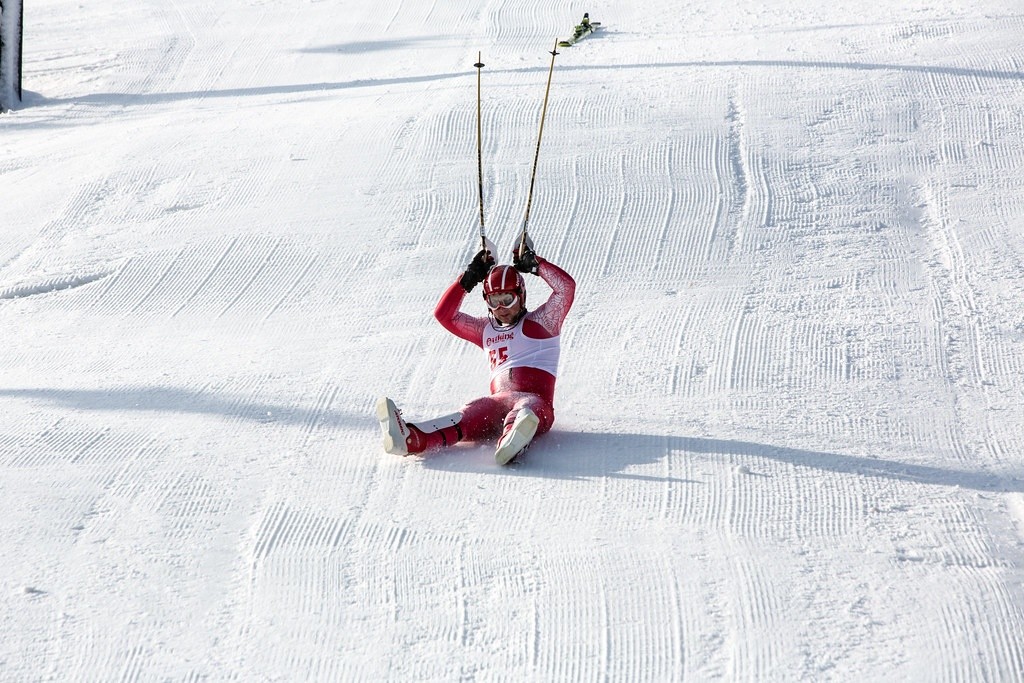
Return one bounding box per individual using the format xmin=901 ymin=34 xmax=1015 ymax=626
xmin=512 ymin=243 xmax=546 ymax=277
xmin=459 ymin=249 xmax=495 ymax=293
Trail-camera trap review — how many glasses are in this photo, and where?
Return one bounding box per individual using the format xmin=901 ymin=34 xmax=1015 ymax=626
xmin=483 ymin=286 xmax=523 ymax=311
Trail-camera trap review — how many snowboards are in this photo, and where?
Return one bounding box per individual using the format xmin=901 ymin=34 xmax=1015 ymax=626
xmin=558 ymin=12 xmax=601 ymax=47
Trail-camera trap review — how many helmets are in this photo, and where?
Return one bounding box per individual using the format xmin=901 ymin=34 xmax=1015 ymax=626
xmin=483 ymin=265 xmax=526 ymax=306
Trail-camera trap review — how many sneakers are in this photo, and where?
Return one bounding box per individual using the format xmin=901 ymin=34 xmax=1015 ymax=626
xmin=495 ymin=407 xmax=541 ymax=467
xmin=377 ymin=396 xmax=410 ymax=457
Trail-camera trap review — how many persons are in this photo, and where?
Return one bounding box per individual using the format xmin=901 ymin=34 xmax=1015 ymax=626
xmin=376 ymin=244 xmax=577 ymax=466
